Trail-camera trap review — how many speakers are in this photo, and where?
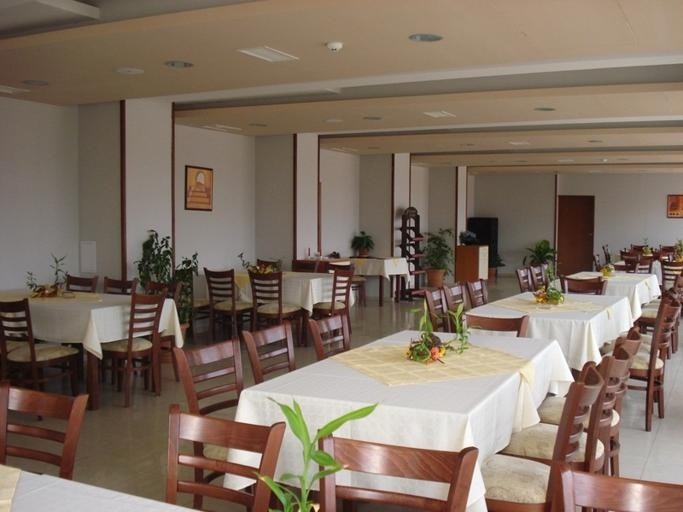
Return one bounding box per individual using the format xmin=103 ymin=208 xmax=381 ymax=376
xmin=468 ymin=216 xmax=498 ymax=266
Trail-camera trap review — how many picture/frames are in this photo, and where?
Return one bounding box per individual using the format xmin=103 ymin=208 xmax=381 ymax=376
xmin=665 ymin=193 xmax=683 ymax=219
xmin=183 ymin=164 xmax=214 ymax=213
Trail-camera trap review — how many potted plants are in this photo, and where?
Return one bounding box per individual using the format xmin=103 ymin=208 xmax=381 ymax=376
xmin=128 ymin=222 xmax=201 ymax=350
xmin=416 ymin=222 xmax=454 ymax=290
xmin=349 ymin=228 xmax=373 ymax=257
xmin=522 ymin=236 xmax=564 ymax=281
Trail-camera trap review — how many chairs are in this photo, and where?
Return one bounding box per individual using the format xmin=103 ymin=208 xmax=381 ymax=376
xmin=202 ymin=260 xmax=366 ymax=347
xmin=517 ymin=265 xmax=552 ymax=294
xmin=423 ymin=278 xmax=492 ymax=333
xmin=314 ymin=430 xmax=478 ymax=512
xmin=102 ymin=279 xmax=186 ymax=408
xmin=64 ymin=274 xmax=103 ymax=295
xmin=3 ymin=385 xmax=90 ymax=479
xmin=480 ymin=348 xmax=682 ymax=512
xmin=172 ymin=314 xmax=350 ymax=510
xmin=598 ymin=275 xmax=682 ymax=433
xmin=0 ymin=300 xmax=85 ymax=391
xmin=166 ymin=402 xmax=287 ymax=512
xmin=594 ymin=243 xmax=682 ymax=273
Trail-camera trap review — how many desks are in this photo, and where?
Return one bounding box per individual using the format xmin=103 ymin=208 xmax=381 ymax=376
xmin=463 ymin=259 xmax=663 ymax=378
xmin=0 ymin=290 xmax=184 ymax=411
xmin=222 ymin=330 xmax=574 ymax=512
xmin=336 ymin=256 xmax=411 ymax=307
